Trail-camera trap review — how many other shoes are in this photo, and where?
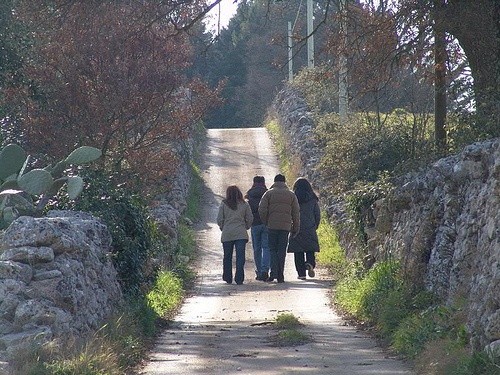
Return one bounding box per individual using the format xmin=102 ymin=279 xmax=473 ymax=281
xmin=255 ymin=272 xmax=274 ymax=281
xmin=235 ymin=281 xmax=243 ymax=285
xmin=277 ymin=276 xmax=284 ymax=283
xmin=226 ymin=280 xmax=232 ymax=284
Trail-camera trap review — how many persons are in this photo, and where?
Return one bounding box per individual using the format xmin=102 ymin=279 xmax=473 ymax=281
xmin=244 ymin=175 xmax=271 ymax=282
xmin=217 ymin=185 xmax=254 ymax=284
xmin=258 ymin=174 xmax=301 ymax=283
xmin=287 ymin=178 xmax=320 ymax=279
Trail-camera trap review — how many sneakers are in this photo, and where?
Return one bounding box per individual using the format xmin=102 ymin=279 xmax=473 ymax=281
xmin=297 ymin=275 xmax=306 ymax=279
xmin=305 ymin=263 xmax=315 ymax=278
